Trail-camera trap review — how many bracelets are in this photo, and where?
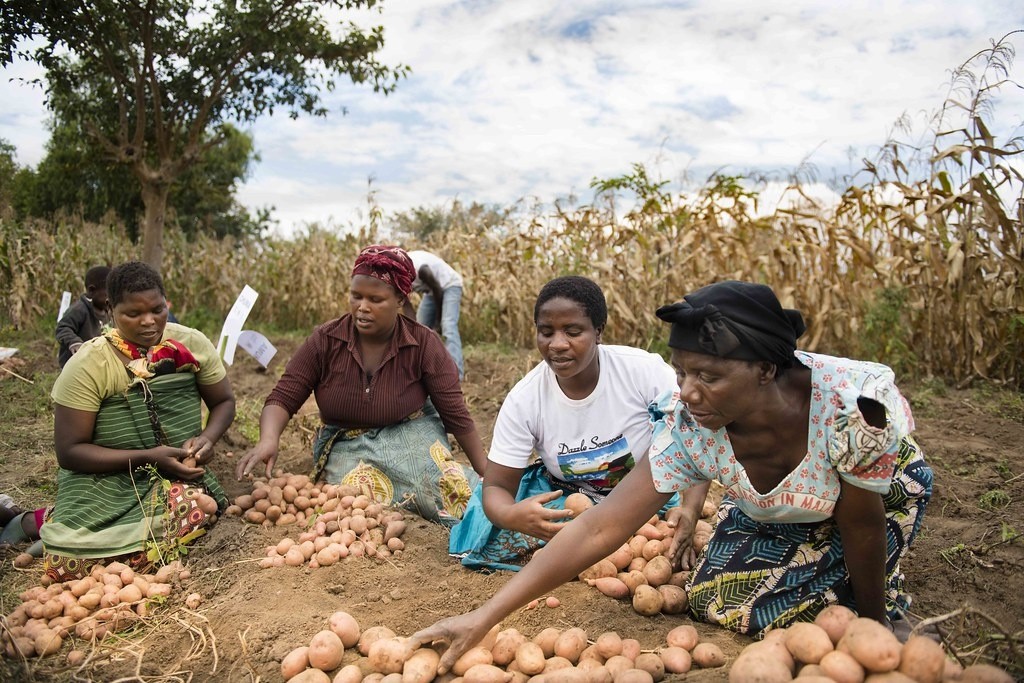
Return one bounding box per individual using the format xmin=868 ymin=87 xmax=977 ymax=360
xmin=887 ymin=620 xmax=895 ymax=633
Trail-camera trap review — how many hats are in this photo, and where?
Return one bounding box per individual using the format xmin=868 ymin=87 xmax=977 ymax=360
xmin=352 ymin=245 xmax=416 ymax=297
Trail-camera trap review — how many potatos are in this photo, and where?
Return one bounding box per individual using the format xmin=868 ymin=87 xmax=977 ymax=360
xmin=728 ymin=605 xmax=1014 ymax=683
xmin=532 ymin=492 xmax=719 ymax=615
xmin=0 ymin=453 xmax=220 ymax=666
xmin=281 ymin=596 xmax=728 ymax=683
xmin=226 ymin=466 xmax=405 ymax=568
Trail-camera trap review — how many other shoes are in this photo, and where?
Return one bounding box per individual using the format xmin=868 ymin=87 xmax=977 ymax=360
xmin=0 ymin=510 xmax=43 ymax=558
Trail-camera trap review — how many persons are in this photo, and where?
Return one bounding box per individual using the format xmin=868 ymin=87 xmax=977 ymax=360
xmin=403 ymin=280 xmax=952 ymax=676
xmin=234 ymin=245 xmax=489 ymax=519
xmin=55 ymin=265 xmax=116 ymax=369
xmin=481 ymin=275 xmax=710 ymax=573
xmin=2 ymin=261 xmax=235 ymax=582
xmin=401 ymin=248 xmax=465 ymax=385
